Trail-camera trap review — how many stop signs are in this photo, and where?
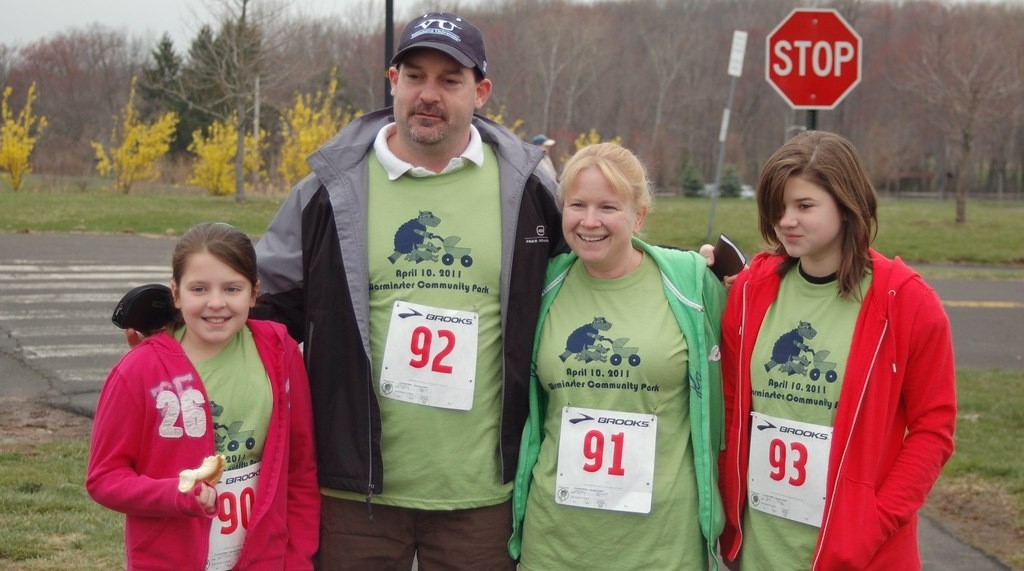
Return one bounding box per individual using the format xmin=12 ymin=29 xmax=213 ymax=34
xmin=765 ymin=8 xmax=864 ymax=109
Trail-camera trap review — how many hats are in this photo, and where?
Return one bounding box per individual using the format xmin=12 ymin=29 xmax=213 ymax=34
xmin=390 ymin=13 xmax=487 ymax=78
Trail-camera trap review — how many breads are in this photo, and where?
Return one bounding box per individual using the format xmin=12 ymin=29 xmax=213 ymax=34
xmin=178 ymin=455 xmax=226 ymax=494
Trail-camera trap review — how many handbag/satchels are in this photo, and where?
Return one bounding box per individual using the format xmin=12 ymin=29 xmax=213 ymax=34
xmin=111 ymin=283 xmax=179 ymax=335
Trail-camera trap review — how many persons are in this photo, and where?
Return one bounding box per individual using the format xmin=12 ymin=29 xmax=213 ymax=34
xmin=722 ymin=130 xmax=958 ymax=571
xmin=508 ymin=142 xmax=727 ymax=570
xmin=121 ymin=11 xmax=747 ymax=571
xmin=86 ymin=219 xmax=319 ymax=570
